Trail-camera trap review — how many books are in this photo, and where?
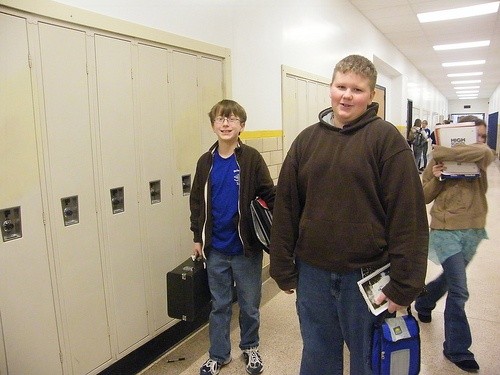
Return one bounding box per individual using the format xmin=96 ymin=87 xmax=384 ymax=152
xmin=433 ymin=120 xmax=482 ymax=180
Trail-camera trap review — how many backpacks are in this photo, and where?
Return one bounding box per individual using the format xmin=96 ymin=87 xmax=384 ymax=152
xmin=411 ymin=128 xmax=422 ymax=145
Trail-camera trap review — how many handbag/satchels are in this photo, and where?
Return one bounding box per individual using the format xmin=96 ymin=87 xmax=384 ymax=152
xmin=247 ymin=196 xmax=272 ymax=253
xmin=370 ymin=306 xmax=421 ymax=375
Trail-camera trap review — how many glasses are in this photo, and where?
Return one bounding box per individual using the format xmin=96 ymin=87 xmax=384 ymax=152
xmin=213 ymin=116 xmax=241 ymax=125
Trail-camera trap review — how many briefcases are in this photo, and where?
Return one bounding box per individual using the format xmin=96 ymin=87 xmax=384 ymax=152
xmin=166 ymin=253 xmax=211 ymax=320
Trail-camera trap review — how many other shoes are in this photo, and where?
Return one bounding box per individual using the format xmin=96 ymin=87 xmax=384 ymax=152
xmin=418 ymin=312 xmax=432 ymax=323
xmin=456 ymin=359 xmax=479 ymax=370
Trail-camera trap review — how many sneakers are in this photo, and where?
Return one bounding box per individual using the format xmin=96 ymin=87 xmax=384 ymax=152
xmin=242 ymin=346 xmax=264 ymax=374
xmin=199 ymin=356 xmax=231 ymax=375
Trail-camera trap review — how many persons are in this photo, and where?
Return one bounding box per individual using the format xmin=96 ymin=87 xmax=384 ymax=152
xmin=414 ymin=115 xmax=496 ymax=371
xmin=408 ymin=118 xmax=453 ymax=174
xmin=189 ymin=100 xmax=277 ymax=375
xmin=271 ymin=54 xmax=429 ymax=375
